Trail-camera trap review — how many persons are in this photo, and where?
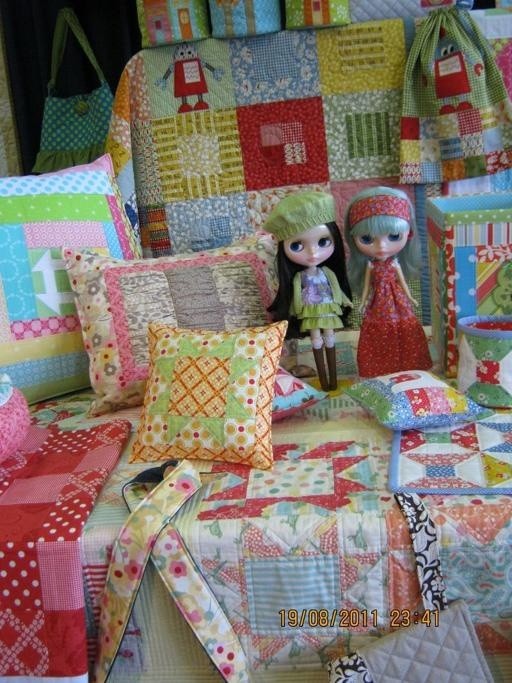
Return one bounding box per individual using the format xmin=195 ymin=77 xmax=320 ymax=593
xmin=261 ymin=191 xmax=352 ymax=392
xmin=343 ymin=185 xmax=433 ymax=377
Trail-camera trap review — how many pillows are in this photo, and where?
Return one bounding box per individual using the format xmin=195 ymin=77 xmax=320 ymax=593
xmin=342 ymin=369 xmax=497 ymax=436
xmin=1 ymin=152 xmax=143 ymax=405
xmin=271 ymin=366 xmax=325 ymax=420
xmin=62 ymin=233 xmax=317 ymax=420
xmin=132 ymin=319 xmax=289 ymax=468
